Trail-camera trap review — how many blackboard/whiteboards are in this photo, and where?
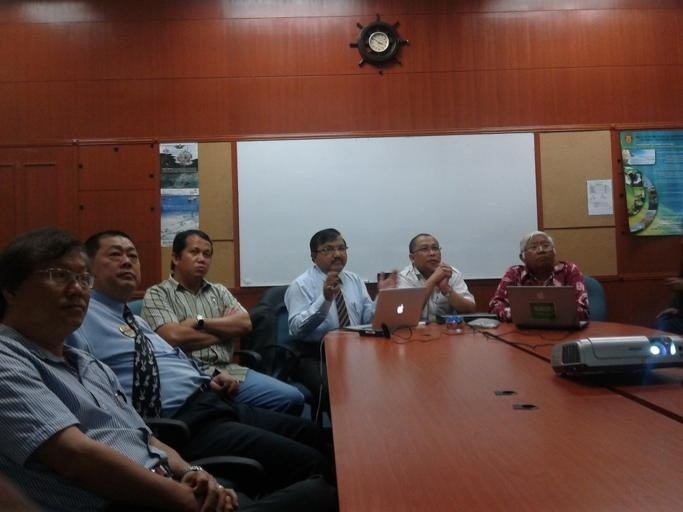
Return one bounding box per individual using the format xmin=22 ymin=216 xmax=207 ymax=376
xmin=231 ymin=131 xmax=544 ymax=291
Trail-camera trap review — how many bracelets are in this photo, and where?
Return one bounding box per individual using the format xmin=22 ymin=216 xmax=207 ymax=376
xmin=179 ymin=466 xmax=207 ymax=474
xmin=442 ymin=286 xmax=454 ymax=297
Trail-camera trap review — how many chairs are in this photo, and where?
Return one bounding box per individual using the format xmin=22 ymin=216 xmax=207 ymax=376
xmin=247 ymin=284 xmax=328 ymax=409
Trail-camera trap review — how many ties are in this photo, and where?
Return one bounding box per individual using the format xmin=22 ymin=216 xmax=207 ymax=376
xmin=329 ymin=275 xmax=352 ymax=329
xmin=123 ymin=303 xmax=166 ymax=421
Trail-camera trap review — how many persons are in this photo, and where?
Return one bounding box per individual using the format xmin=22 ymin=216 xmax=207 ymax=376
xmin=392 ymin=234 xmax=477 ymax=322
xmin=489 ymin=232 xmax=589 ymax=322
xmin=142 ymin=228 xmax=308 ymax=415
xmin=68 ymin=230 xmax=336 ymax=486
xmin=283 ymin=226 xmax=401 ymax=341
xmin=654 ymin=270 xmax=682 ymax=333
xmin=0 ymin=228 xmax=338 ymax=510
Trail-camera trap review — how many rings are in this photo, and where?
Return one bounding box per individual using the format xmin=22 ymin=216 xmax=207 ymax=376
xmin=326 ymin=283 xmax=334 ymax=291
xmin=214 ymin=482 xmax=225 ymax=491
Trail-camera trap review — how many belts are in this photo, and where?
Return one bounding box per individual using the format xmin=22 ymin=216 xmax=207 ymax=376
xmin=150 ymin=465 xmax=171 ymax=478
xmin=176 ymin=383 xmax=210 ymax=417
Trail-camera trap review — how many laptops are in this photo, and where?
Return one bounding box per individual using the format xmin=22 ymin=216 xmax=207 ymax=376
xmin=506 ymin=285 xmax=588 ymax=330
xmin=343 ymin=286 xmax=427 ymax=331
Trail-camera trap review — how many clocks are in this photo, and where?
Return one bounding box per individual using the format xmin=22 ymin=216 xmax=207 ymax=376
xmin=350 ymin=11 xmax=410 ymax=76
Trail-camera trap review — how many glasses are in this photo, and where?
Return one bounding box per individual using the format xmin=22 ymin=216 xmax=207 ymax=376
xmin=521 ymin=242 xmax=554 ymax=252
xmin=410 ymin=244 xmax=442 ymax=252
xmin=312 ymin=246 xmax=349 ymax=254
xmin=27 ymin=267 xmax=95 ymax=289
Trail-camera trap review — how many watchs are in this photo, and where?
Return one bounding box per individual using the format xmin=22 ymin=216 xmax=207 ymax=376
xmin=195 ymin=314 xmax=205 ymax=327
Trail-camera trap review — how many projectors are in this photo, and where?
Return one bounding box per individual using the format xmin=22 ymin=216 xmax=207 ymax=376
xmin=550 ymin=333 xmax=682 ymax=378
